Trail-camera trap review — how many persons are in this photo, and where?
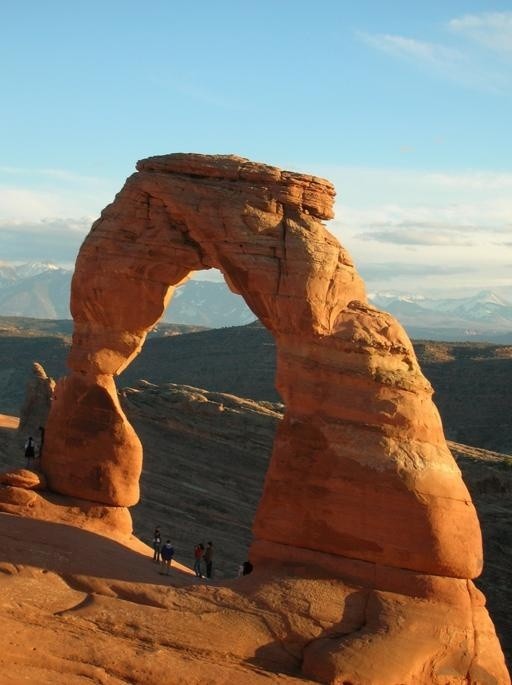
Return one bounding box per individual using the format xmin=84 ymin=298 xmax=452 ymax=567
xmin=160 ymin=540 xmax=174 ymax=576
xmin=203 ymin=542 xmax=213 ymax=580
xmin=25 ymin=436 xmax=36 ymax=468
xmin=152 ymin=525 xmax=161 ymax=562
xmin=38 ymin=425 xmax=45 ymax=456
xmin=194 ymin=544 xmax=205 ymax=577
